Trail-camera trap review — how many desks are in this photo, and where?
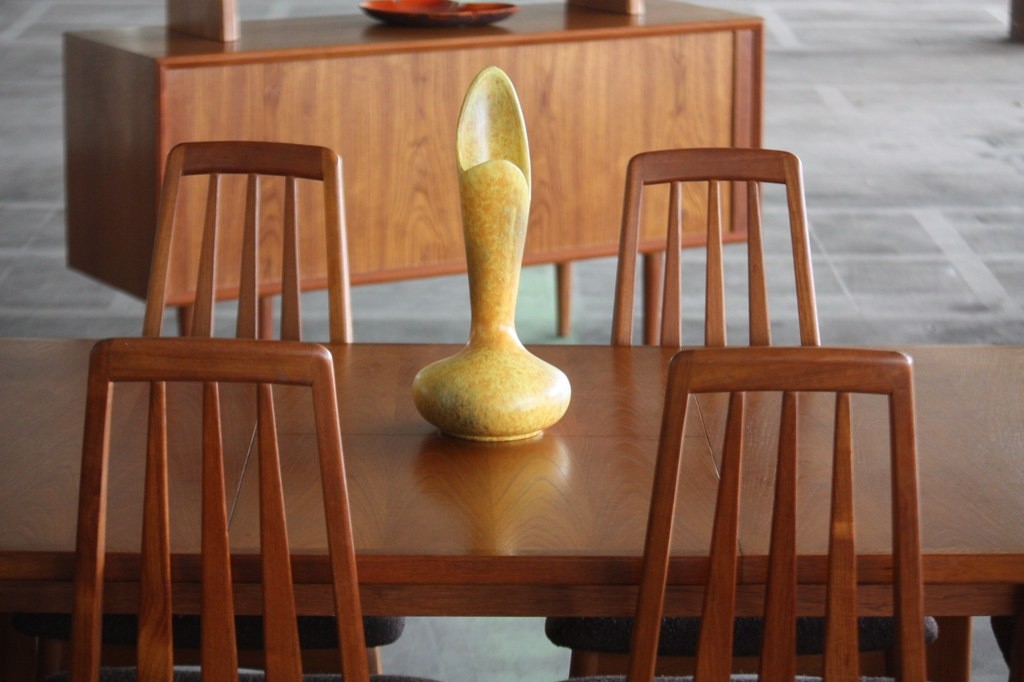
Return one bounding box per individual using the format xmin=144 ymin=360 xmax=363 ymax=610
xmin=0 ymin=335 xmax=1024 ymax=682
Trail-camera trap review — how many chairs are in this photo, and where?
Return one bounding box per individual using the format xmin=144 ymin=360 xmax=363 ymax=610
xmin=15 ymin=141 xmax=438 ymax=682
xmin=546 ymin=147 xmax=927 ymax=682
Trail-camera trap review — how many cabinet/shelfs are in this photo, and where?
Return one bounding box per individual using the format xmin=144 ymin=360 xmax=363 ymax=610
xmin=64 ymin=0 xmax=765 ymax=338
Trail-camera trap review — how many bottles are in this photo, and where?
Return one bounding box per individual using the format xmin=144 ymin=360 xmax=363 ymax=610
xmin=412 ymin=64 xmax=572 ymax=442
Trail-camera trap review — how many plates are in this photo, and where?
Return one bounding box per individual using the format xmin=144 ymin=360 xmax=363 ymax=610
xmin=358 ymin=0 xmax=519 ymax=26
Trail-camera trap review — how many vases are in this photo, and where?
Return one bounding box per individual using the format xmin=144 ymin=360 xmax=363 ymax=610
xmin=412 ymin=67 xmax=573 ymax=443
